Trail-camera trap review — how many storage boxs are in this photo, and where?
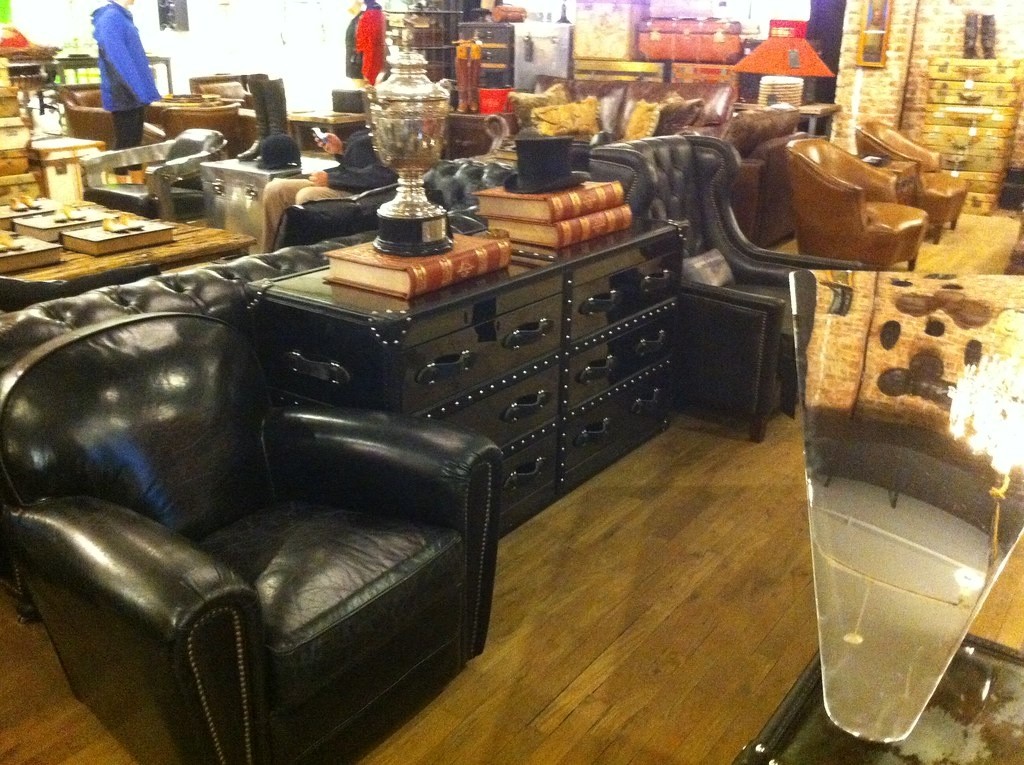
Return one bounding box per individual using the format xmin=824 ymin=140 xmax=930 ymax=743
xmin=32 ymin=138 xmax=106 ymax=206
xmin=0 ymin=86 xmax=21 ymax=118
xmin=671 ymin=62 xmax=740 ymax=101
xmin=640 ymin=17 xmax=742 ymax=63
xmin=0 ymin=57 xmax=13 ymax=87
xmin=573 ymin=0 xmax=650 ymax=62
xmin=0 ymin=126 xmax=31 ymax=175
xmin=573 ymin=60 xmax=666 ymax=83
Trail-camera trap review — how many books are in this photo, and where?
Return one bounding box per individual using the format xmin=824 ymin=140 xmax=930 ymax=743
xmin=323 ymin=231 xmax=510 ymax=300
xmin=473 ymin=182 xmax=633 ymax=248
xmin=0 ymin=203 xmax=176 ymax=275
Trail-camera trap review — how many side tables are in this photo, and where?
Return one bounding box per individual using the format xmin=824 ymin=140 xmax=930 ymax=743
xmin=736 ymin=620 xmax=1024 ymax=765
xmin=864 ymin=154 xmax=917 ymax=205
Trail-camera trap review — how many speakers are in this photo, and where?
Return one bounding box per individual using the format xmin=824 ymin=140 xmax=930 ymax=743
xmin=157 ymin=0 xmax=189 ymax=33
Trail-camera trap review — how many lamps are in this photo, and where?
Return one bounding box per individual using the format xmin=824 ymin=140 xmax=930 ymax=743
xmin=733 ymin=36 xmax=835 ymax=105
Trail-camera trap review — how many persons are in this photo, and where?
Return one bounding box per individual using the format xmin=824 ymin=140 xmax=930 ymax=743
xmin=356 ymin=0 xmax=386 ymax=84
xmin=347 ymin=0 xmax=364 ymax=89
xmin=92 ymin=0 xmax=162 ymax=173
xmin=264 ymin=129 xmax=396 ymax=252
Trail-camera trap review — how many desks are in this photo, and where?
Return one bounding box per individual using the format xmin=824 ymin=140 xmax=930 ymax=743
xmin=10 ymin=62 xmax=45 ymax=115
xmin=146 ymin=100 xmax=232 ymax=129
xmin=0 ymin=201 xmax=257 ymax=297
xmin=57 ymin=58 xmax=173 ymax=99
xmin=732 ymin=102 xmax=843 ymax=133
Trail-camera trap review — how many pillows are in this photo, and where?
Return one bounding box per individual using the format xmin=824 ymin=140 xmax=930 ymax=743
xmin=625 ymin=99 xmax=661 ymax=140
xmin=508 ymin=83 xmax=571 ymax=128
xmin=531 ymin=95 xmax=601 ymax=135
xmin=657 ymin=92 xmax=705 ymax=135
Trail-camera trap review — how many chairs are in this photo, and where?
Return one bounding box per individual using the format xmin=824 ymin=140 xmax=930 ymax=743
xmin=68 ymin=91 xmax=117 ymax=144
xmin=161 ymin=103 xmax=241 ymax=142
xmin=78 ymin=130 xmax=227 ymax=222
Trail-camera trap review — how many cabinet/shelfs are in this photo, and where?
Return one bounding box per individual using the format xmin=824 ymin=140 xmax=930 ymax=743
xmin=243 ymin=219 xmax=690 ymax=539
xmin=200 ymin=157 xmax=338 ymax=254
xmin=515 ymin=23 xmax=573 ymax=89
xmin=458 ymin=22 xmax=512 ymax=87
xmin=291 ymin=110 xmax=366 ymax=158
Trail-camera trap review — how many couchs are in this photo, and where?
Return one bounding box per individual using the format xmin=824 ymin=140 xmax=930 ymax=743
xmin=0 ymin=312 xmax=502 ymax=765
xmin=479 ymin=74 xmax=739 ymax=140
xmin=587 ymin=133 xmax=862 ymax=440
xmin=856 ymin=122 xmax=969 ymax=242
xmin=0 ymin=158 xmax=515 ymax=375
xmin=191 ymin=74 xmax=254 ymax=108
xmin=724 ymin=106 xmax=808 ymax=246
xmin=784 ymin=138 xmax=927 ymax=270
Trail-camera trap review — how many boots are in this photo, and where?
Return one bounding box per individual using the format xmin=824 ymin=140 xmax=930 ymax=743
xmin=454 ymin=58 xmax=469 ymax=114
xmin=468 ymin=58 xmax=481 ymax=113
xmin=237 ymin=74 xmax=269 ymax=162
xmin=264 ymin=78 xmax=289 ymax=136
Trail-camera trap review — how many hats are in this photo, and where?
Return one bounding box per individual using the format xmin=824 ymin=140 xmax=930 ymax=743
xmin=323 ymin=136 xmax=401 ymax=189
xmin=503 ymin=137 xmax=586 ymax=194
xmin=256 ymin=133 xmax=302 ymax=169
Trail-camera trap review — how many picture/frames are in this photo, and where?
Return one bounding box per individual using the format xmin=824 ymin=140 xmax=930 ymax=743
xmin=857 ymin=0 xmax=895 ymax=69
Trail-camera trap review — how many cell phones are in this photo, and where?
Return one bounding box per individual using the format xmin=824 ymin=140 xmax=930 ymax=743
xmin=311 ymin=127 xmax=328 ymax=143
